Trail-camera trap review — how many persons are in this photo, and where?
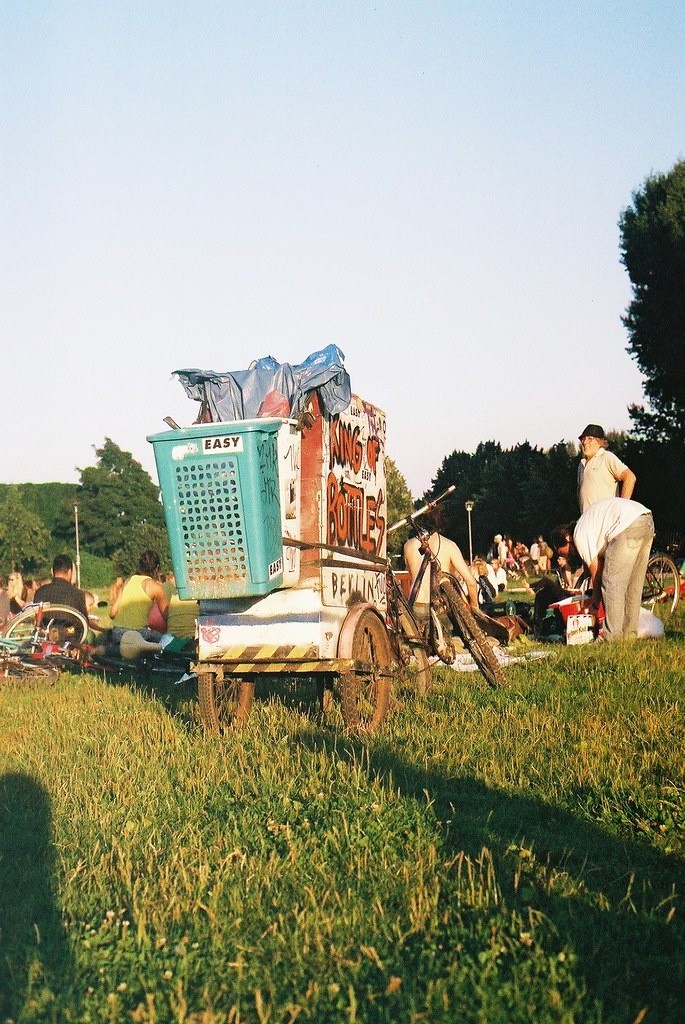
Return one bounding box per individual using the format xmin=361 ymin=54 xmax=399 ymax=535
xmin=458 ymin=555 xmax=507 ymax=604
xmin=519 ymin=557 xmax=572 ymax=595
xmin=404 ymin=500 xmax=478 ymax=622
xmin=486 ymin=534 xmax=551 ymax=581
xmin=7 ymin=571 xmax=28 ymax=623
xmin=33 ymin=554 xmax=106 ymax=645
xmin=91 ymin=549 xmax=172 ymax=658
xmin=575 ymin=424 xmax=636 ymax=516
xmin=551 ymin=494 xmax=655 ymax=646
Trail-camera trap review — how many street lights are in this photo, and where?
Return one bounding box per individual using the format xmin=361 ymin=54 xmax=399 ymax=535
xmin=70 ymin=498 xmax=82 ymax=591
xmin=464 ymin=500 xmax=475 ymax=566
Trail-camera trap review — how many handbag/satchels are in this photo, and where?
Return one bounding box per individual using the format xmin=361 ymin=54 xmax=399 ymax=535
xmin=545 ymin=547 xmax=553 ymax=559
xmin=491 ymin=616 xmax=529 ymax=639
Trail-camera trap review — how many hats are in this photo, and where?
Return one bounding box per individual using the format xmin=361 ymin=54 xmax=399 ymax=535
xmin=495 ymin=534 xmax=502 ymax=540
xmin=578 ymin=424 xmax=607 ymax=440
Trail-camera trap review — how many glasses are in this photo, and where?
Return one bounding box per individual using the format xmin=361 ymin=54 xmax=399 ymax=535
xmin=581 ymin=438 xmax=594 ymax=442
xmin=8 ymin=578 xmax=16 ymax=581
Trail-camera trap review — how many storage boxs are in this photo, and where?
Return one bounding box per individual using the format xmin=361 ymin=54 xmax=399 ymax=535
xmin=548 ymin=593 xmax=607 ymax=647
xmin=145 ymin=419 xmax=284 ymax=601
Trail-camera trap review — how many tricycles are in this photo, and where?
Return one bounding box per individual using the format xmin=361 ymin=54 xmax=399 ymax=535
xmin=189 ymin=483 xmax=507 ymax=739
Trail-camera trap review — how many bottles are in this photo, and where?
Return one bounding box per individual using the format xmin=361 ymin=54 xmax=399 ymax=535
xmin=506 ymin=598 xmax=516 ymax=615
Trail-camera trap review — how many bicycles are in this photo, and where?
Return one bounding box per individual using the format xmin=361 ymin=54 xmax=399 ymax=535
xmin=576 ymin=550 xmax=681 ymax=629
xmin=0 ymin=600 xmax=189 ymax=690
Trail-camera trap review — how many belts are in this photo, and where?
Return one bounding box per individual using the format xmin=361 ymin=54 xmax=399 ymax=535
xmin=413 ymin=602 xmax=430 ymax=607
